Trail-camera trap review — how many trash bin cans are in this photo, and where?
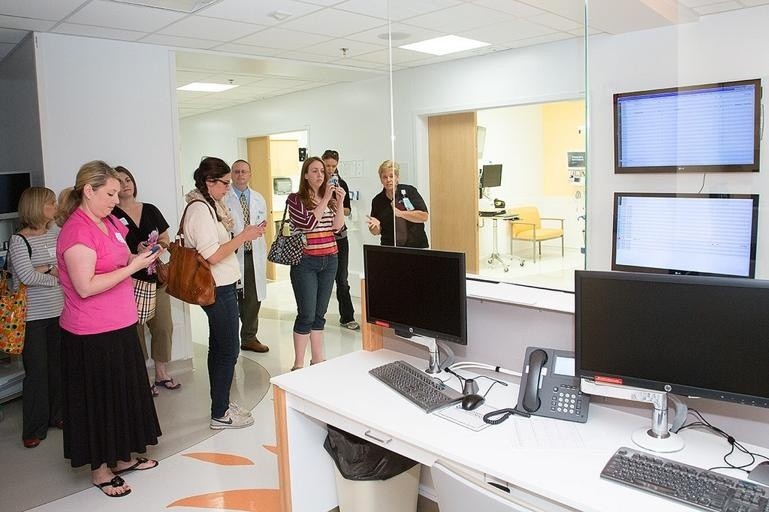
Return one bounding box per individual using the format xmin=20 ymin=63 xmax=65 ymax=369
xmin=323 ymin=427 xmax=422 ymax=511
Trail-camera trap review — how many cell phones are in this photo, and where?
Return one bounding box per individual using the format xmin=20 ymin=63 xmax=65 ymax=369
xmin=147 ymin=245 xmax=159 ymax=257
xmin=255 ymin=220 xmax=267 ymax=228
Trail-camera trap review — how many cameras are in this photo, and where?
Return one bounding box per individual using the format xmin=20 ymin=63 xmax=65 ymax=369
xmin=330 ymin=177 xmax=341 ymax=188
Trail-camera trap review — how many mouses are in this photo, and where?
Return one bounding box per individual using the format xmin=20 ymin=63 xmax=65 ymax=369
xmin=462 ymin=394 xmax=485 ymax=411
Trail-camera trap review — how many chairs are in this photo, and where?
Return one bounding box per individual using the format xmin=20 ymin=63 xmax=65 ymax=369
xmin=506 ymin=204 xmax=566 ymax=266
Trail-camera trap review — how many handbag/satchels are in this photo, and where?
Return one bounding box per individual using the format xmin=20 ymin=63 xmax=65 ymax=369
xmin=129 ymin=275 xmax=157 ymax=326
xmin=0 ymin=269 xmax=27 ymax=358
xmin=266 ymin=231 xmax=306 ymax=267
xmin=165 ymin=242 xmax=216 ymax=306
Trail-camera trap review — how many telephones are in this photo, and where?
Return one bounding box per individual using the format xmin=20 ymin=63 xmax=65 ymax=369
xmin=515 ymin=346 xmax=590 ymax=423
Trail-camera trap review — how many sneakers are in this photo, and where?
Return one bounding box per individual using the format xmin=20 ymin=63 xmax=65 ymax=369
xmin=228 ymin=400 xmax=251 ymax=417
xmin=210 ymin=409 xmax=255 ymax=430
xmin=241 ymin=337 xmax=270 ymax=353
xmin=340 ymin=319 xmax=360 ymax=330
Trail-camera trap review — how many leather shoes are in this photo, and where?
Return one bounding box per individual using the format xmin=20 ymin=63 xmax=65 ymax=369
xmin=23 ymin=439 xmax=41 ymax=448
xmin=51 ymin=418 xmax=65 ymax=430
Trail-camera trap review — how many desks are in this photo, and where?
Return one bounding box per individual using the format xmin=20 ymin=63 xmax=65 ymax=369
xmin=265 ymin=342 xmax=769 ymax=511
xmin=478 ymin=207 xmax=526 ymax=273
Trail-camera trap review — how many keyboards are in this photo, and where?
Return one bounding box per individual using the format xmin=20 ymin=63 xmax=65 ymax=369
xmin=370 ymin=359 xmax=467 ymax=414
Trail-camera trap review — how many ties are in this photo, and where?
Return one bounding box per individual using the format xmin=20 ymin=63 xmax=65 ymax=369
xmin=240 ymin=193 xmax=252 ymax=251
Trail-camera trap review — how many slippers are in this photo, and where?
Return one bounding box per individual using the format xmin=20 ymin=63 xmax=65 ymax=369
xmin=150 ymin=384 xmax=159 ymax=398
xmin=112 ymin=456 xmax=160 ymax=475
xmin=92 ymin=474 xmax=132 ymax=498
xmin=154 ymin=376 xmax=182 ymax=390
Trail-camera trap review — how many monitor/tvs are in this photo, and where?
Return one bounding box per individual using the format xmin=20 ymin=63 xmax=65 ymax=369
xmin=610 ymin=190 xmax=760 ymax=279
xmin=567 ymin=151 xmax=586 ymax=171
xmin=361 ymin=243 xmax=469 ymax=347
xmin=611 ymin=78 xmax=762 ymax=174
xmin=573 ymin=268 xmax=769 ymax=409
xmin=598 ymin=446 xmax=769 ymax=512
xmin=481 ymin=163 xmax=503 ymax=188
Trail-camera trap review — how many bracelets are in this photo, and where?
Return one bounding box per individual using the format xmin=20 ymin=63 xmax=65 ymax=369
xmin=47 ymin=263 xmax=55 ymax=274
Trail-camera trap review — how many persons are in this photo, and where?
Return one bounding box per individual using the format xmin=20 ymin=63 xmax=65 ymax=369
xmin=181 ymin=156 xmax=266 ymax=429
xmin=365 ymin=160 xmax=430 ymax=249
xmin=9 ymin=186 xmax=64 ymax=449
xmin=219 ymin=159 xmax=270 ymax=353
xmin=319 ymin=149 xmax=360 ymax=330
xmin=286 ymin=157 xmax=346 ymax=372
xmin=53 ymin=160 xmax=167 ymax=498
xmin=113 ymin=165 xmax=182 ymax=397
